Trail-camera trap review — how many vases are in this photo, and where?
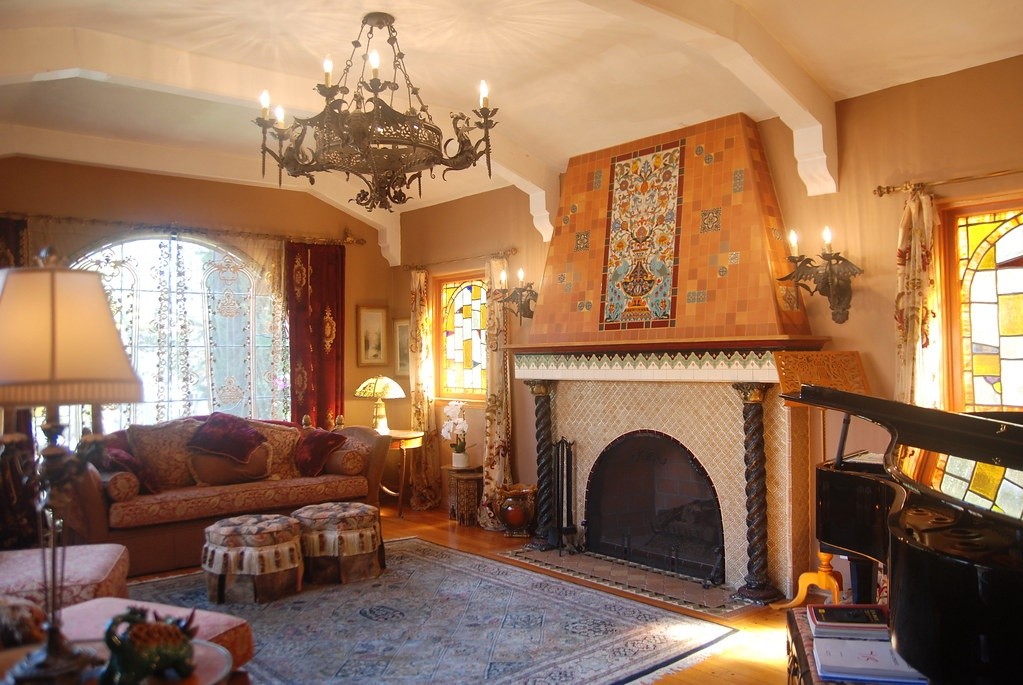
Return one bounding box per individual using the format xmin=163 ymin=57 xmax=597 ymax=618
xmin=492 ymin=484 xmax=537 ymax=537
xmin=452 ymin=452 xmax=471 ymax=466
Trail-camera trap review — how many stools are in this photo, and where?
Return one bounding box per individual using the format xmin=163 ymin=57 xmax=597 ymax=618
xmin=290 ymin=501 xmax=386 ymax=586
xmin=201 ymin=514 xmax=304 ymax=603
xmin=453 ymin=472 xmax=483 ymax=526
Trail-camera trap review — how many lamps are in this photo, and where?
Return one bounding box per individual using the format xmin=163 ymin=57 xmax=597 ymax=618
xmin=493 ymin=267 xmax=538 ymax=327
xmin=249 ymin=12 xmax=500 ymax=214
xmin=776 ymin=226 xmax=864 ymax=324
xmin=354 ymin=374 xmax=406 ymax=434
xmin=0 ymin=244 xmax=140 ymax=685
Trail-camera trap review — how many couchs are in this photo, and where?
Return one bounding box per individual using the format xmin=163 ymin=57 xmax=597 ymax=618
xmin=75 ymin=413 xmax=390 ymax=578
xmin=0 ymin=543 xmax=253 ymax=685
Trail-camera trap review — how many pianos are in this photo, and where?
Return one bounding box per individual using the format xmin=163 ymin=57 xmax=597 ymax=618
xmin=778 ymin=383 xmax=1023 ymax=685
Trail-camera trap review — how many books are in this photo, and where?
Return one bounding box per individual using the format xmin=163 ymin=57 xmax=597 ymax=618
xmin=807 ymin=604 xmax=890 ymax=640
xmin=812 ymin=638 xmax=930 ymax=685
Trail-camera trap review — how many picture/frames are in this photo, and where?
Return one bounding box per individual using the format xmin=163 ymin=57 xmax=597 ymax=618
xmin=356 ymin=304 xmax=390 ymax=367
xmin=393 ymin=318 xmax=409 ymax=376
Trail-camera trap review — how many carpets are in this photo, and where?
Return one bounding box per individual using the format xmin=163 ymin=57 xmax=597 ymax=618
xmin=495 ymin=546 xmax=763 ymax=620
xmin=129 ymin=536 xmax=740 ymax=684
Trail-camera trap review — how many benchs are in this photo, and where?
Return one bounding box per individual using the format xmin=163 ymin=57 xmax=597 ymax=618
xmin=786 ymin=607 xmax=870 ymax=685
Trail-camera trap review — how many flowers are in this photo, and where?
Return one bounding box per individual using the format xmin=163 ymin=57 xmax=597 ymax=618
xmin=440 ymin=400 xmax=478 ymax=453
xmin=0 ymin=432 xmax=117 ymax=494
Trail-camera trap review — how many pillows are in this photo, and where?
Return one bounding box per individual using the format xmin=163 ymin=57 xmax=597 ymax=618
xmin=187 ymin=412 xmax=269 ymax=464
xmin=101 ymin=471 xmax=139 ymax=502
xmin=326 ymin=450 xmax=364 ymax=475
xmin=295 ymin=428 xmax=347 ymax=476
xmin=188 ymin=442 xmax=272 ymax=487
xmin=103 ymin=447 xmax=160 ymax=492
xmin=343 ymin=435 xmax=372 ymax=477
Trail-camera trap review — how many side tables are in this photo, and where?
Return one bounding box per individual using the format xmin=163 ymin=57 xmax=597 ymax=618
xmin=380 ymin=430 xmax=425 ymax=517
xmin=1 ymin=638 xmax=233 ymax=685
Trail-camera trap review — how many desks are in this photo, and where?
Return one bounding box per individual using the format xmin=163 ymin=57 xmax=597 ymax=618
xmin=441 ymin=464 xmax=483 ymax=520
